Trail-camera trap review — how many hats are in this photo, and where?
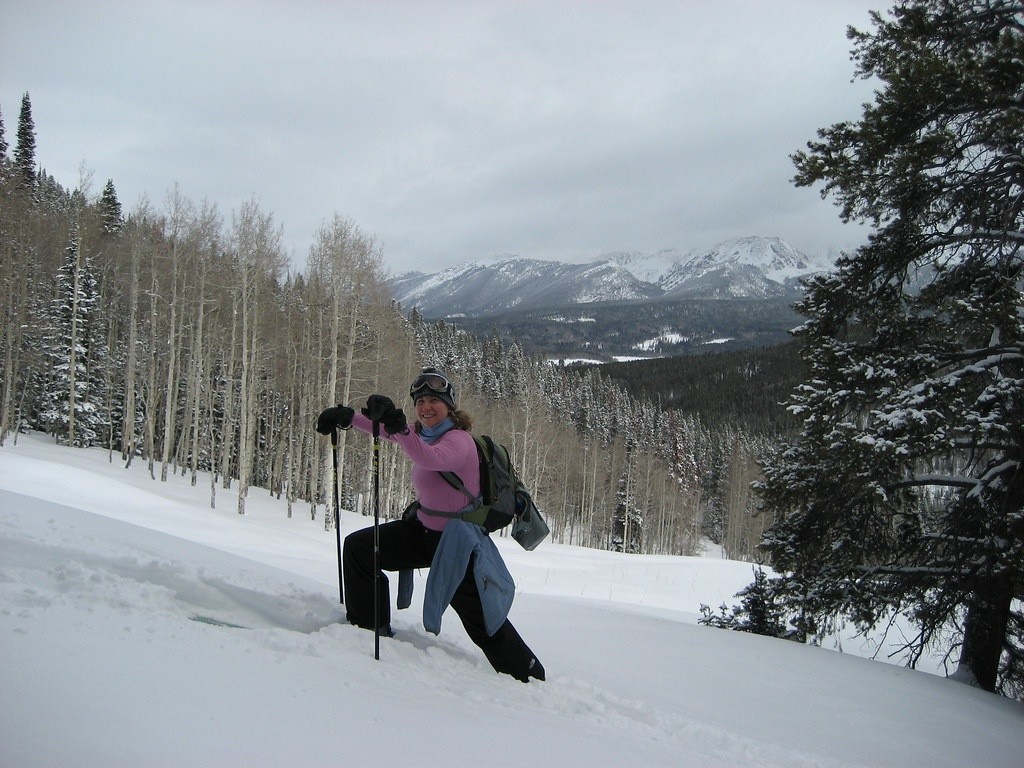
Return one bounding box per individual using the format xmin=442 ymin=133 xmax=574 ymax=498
xmin=413 ymin=366 xmax=456 ymax=410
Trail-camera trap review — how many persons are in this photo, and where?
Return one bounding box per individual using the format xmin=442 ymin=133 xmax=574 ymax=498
xmin=316 ymin=367 xmax=549 ymax=685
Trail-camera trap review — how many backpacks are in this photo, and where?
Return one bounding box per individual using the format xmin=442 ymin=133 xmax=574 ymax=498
xmin=434 ymin=428 xmax=515 ymax=532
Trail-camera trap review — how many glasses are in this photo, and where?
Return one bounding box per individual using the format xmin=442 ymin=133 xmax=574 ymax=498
xmin=410 ymin=373 xmax=450 ymax=393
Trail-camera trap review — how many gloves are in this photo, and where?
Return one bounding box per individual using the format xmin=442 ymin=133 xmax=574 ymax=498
xmin=317 ymin=404 xmax=354 ymax=435
xmin=367 ymin=394 xmax=406 ymax=434
